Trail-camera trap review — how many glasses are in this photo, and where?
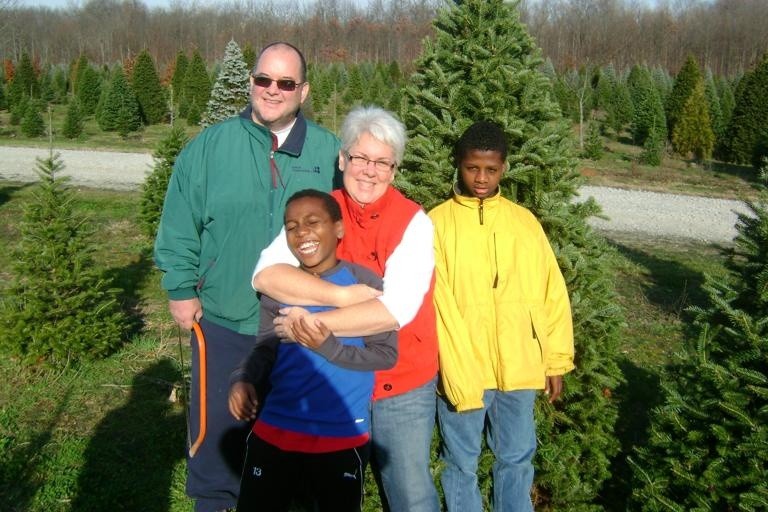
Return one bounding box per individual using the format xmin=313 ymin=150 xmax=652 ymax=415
xmin=252 ymin=74 xmax=304 ymax=92
xmin=345 ymin=151 xmax=395 ymax=173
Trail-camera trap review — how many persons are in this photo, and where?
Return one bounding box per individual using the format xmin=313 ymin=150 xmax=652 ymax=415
xmin=425 ymin=120 xmax=577 ymax=512
xmin=153 ymin=43 xmax=346 ymax=510
xmin=251 ymin=106 xmax=441 ymax=512
xmin=227 ymin=188 xmax=399 ymax=511
